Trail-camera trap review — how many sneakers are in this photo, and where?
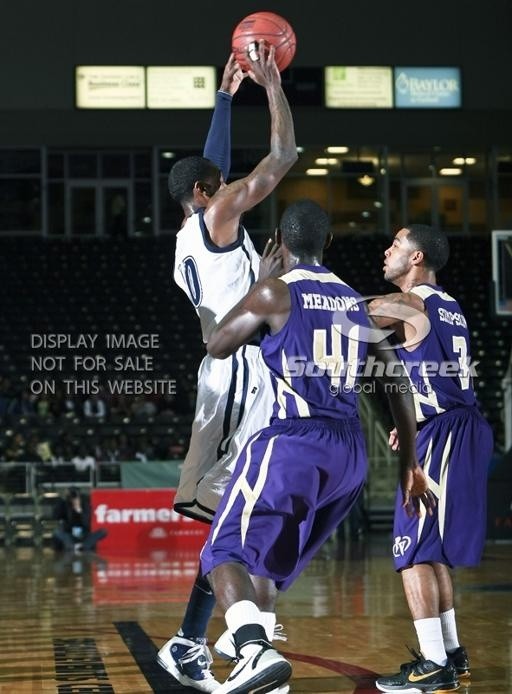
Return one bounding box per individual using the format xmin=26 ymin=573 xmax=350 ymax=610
xmin=398 ymin=646 xmax=471 ymax=678
xmin=375 ymin=658 xmax=461 ymax=693
xmin=155 ymin=628 xmax=223 ymax=693
xmin=212 ymin=628 xmax=292 ymax=694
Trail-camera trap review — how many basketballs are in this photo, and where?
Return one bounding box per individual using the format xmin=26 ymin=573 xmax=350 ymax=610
xmin=232 ymin=12 xmax=296 ymax=73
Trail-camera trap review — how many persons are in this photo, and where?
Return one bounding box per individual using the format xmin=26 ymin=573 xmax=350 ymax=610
xmin=366 ymin=223 xmax=497 ymax=694
xmin=153 ymin=38 xmax=301 ymax=692
xmin=1 ymin=237 xmax=511 ymax=554
xmin=198 ymin=197 xmax=439 ymax=693
